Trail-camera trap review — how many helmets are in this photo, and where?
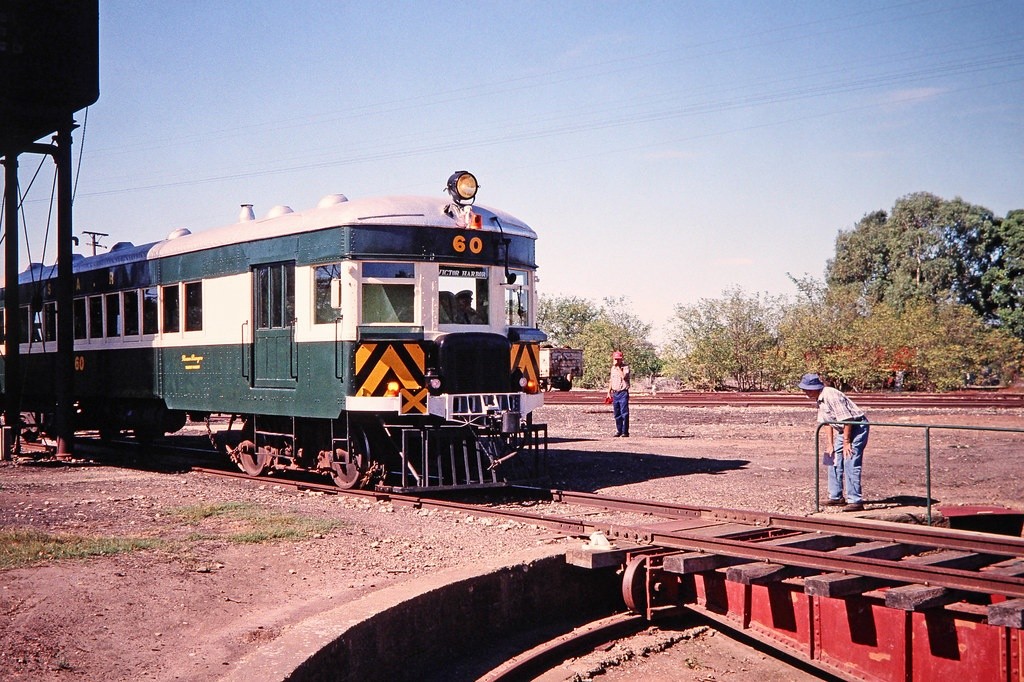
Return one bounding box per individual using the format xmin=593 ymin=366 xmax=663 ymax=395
xmin=613 ymin=352 xmax=622 ymax=359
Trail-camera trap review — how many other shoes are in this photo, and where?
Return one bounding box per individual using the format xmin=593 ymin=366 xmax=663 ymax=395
xmin=622 ymin=433 xmax=629 ymax=437
xmin=843 ymin=503 xmax=864 ymax=511
xmin=613 ymin=431 xmax=622 ymax=437
xmin=819 ymin=497 xmax=846 ymax=506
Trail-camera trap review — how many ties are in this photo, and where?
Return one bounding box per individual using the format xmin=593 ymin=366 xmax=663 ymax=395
xmin=464 ymin=313 xmax=471 ymax=324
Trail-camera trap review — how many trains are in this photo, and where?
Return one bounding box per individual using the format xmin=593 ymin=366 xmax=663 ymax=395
xmin=0 ymin=169 xmax=548 ymax=495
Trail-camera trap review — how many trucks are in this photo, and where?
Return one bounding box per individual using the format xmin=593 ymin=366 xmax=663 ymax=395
xmin=540 ymin=345 xmax=583 ymax=391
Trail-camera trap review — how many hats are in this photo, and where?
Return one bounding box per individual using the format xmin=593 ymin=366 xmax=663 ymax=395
xmin=798 ymin=374 xmax=824 ymax=390
xmin=455 ymin=290 xmax=473 ymax=300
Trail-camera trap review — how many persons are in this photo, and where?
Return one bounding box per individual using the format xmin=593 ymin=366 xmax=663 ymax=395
xmin=799 ymin=374 xmax=869 ymax=512
xmin=607 ymin=352 xmax=631 ymax=437
xmin=447 ymin=290 xmax=484 ymax=325
